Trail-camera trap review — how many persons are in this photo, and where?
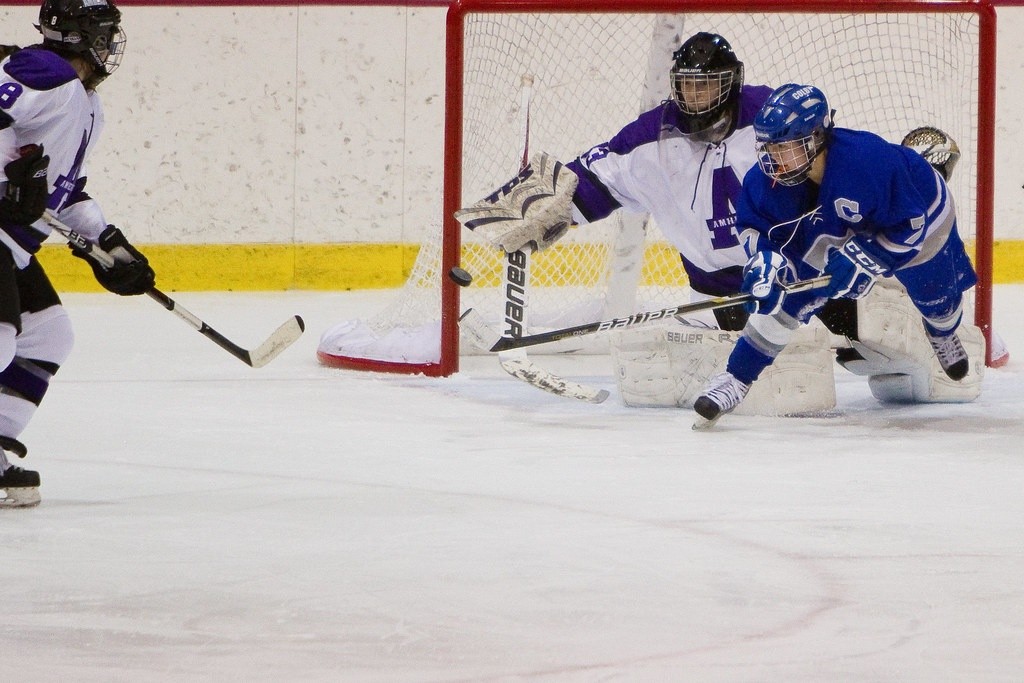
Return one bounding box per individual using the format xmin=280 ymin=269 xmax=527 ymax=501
xmin=453 ymin=32 xmax=986 ymax=416
xmin=692 ymin=83 xmax=979 ymax=430
xmin=0 ymin=0 xmax=155 ymax=508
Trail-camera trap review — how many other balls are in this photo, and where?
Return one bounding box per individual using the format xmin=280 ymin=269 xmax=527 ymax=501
xmin=448 ymin=266 xmax=473 ymax=287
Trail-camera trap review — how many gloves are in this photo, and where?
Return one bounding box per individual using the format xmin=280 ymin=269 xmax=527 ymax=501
xmin=811 ymin=236 xmax=891 ymax=301
xmin=743 ymin=247 xmax=799 ymax=316
xmin=0 ymin=140 xmax=50 ymax=224
xmin=69 ymin=223 xmax=155 ymax=296
xmin=451 ymin=149 xmax=580 ymax=256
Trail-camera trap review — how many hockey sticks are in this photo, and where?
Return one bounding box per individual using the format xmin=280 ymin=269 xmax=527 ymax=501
xmin=456 ymin=274 xmax=836 ymax=355
xmin=497 ymin=70 xmax=610 ymax=406
xmin=1 ymin=182 xmax=307 ymax=371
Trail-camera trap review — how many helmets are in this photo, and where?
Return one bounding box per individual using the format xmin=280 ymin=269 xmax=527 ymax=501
xmin=30 ymin=0 xmax=126 ymax=91
xmin=670 ymin=31 xmax=743 ymax=135
xmin=753 ymin=83 xmax=836 ymax=188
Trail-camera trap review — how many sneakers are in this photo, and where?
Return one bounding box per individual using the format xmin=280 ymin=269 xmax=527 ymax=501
xmin=921 ymin=316 xmax=969 ymax=381
xmin=0 ymin=447 xmax=41 ymax=507
xmin=691 ymin=371 xmax=752 ymax=430
